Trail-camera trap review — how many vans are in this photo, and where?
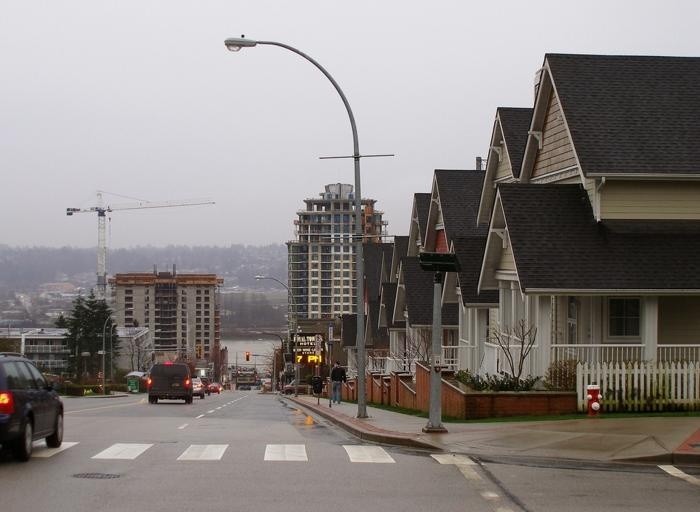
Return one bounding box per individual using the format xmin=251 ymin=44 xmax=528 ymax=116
xmin=146 ymin=359 xmax=194 ymax=406
xmin=283 ymin=377 xmax=307 ymax=396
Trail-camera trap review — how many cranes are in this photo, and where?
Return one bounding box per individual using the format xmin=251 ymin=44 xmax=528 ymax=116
xmin=64 ymin=186 xmax=217 ymax=304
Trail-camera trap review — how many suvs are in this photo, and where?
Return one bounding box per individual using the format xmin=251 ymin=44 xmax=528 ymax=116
xmin=0 ymin=349 xmax=66 ymax=463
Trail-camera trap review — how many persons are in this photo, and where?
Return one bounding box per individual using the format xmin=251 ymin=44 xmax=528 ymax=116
xmin=329 ymin=360 xmax=347 ymax=404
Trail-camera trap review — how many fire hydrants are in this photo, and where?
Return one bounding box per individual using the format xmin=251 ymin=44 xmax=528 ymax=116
xmin=584 ymin=380 xmax=604 ymax=419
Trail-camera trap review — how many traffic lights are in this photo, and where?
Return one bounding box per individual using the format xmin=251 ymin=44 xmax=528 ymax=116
xmin=246 ymin=351 xmax=250 ymax=362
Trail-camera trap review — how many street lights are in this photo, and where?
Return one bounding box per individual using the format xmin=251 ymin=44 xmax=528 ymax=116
xmin=256 ymin=337 xmax=276 ymax=394
xmin=253 ymin=274 xmax=301 ymax=398
xmin=225 ymin=34 xmax=373 ymax=421
xmin=102 ymin=307 xmax=138 ymax=396
xmin=108 ymin=322 xmax=117 ymax=385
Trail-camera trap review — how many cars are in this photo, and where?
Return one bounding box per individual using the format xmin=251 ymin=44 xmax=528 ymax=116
xmin=239 ymin=385 xmax=252 ymax=390
xmin=191 ymin=375 xmax=222 ymax=399
xmin=264 ymin=380 xmax=271 ymax=391
